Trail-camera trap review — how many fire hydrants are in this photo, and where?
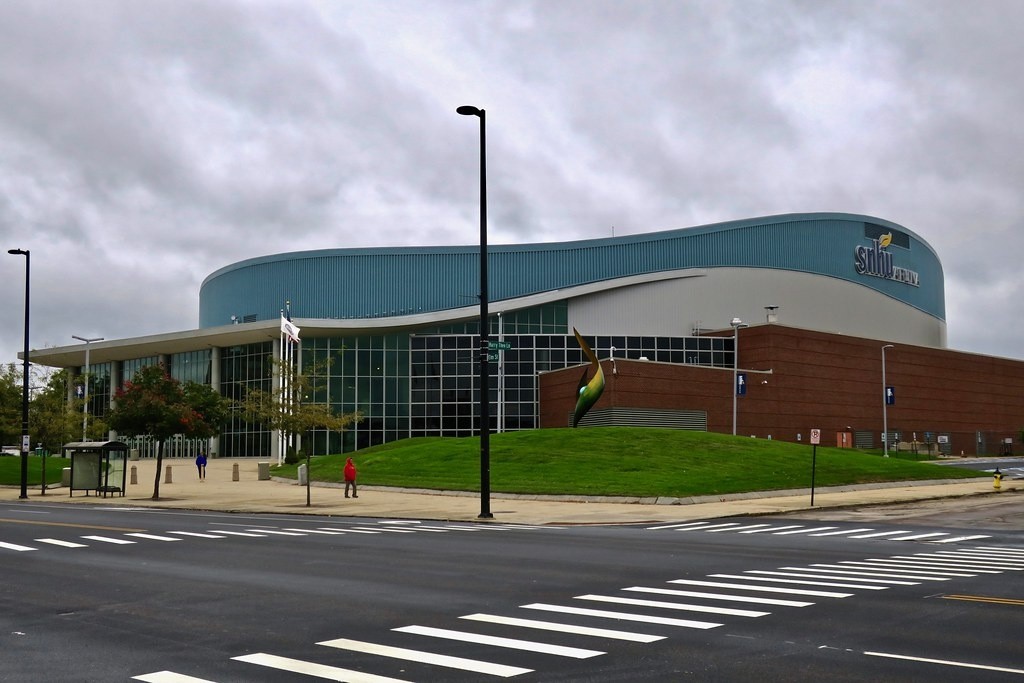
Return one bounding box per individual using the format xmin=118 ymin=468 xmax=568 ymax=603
xmin=994 ymin=465 xmax=1004 ymax=493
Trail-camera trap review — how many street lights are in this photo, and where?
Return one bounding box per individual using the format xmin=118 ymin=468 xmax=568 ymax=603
xmin=8 ymin=248 xmax=32 ymax=499
xmin=457 ymin=104 xmax=494 ymax=517
xmin=71 ymin=334 xmax=105 ymax=453
xmin=881 ymin=344 xmax=896 ymax=457
xmin=731 ymin=322 xmax=750 ymax=435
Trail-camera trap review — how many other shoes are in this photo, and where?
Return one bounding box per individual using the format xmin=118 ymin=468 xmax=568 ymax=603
xmin=345 ymin=496 xmax=350 ymax=498
xmin=352 ymin=494 xmax=358 ymax=498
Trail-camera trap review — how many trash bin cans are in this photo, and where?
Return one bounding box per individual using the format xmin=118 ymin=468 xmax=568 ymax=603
xmin=62 ymin=467 xmax=70 ymax=487
xmin=258 ymin=462 xmax=269 ymax=480
xmin=130 ymin=449 xmax=139 ymax=461
xmin=35 ymin=447 xmax=48 ymax=457
xmin=297 ymin=464 xmax=312 ymax=486
xmin=836 ymin=431 xmax=852 ymax=448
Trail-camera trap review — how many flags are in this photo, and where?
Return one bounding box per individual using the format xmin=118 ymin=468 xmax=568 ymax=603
xmin=282 ymin=310 xmax=301 ymax=344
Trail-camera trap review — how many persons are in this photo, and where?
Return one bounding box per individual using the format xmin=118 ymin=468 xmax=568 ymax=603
xmin=344 ymin=457 xmax=359 ymax=498
xmin=196 ymin=452 xmax=207 ymax=482
